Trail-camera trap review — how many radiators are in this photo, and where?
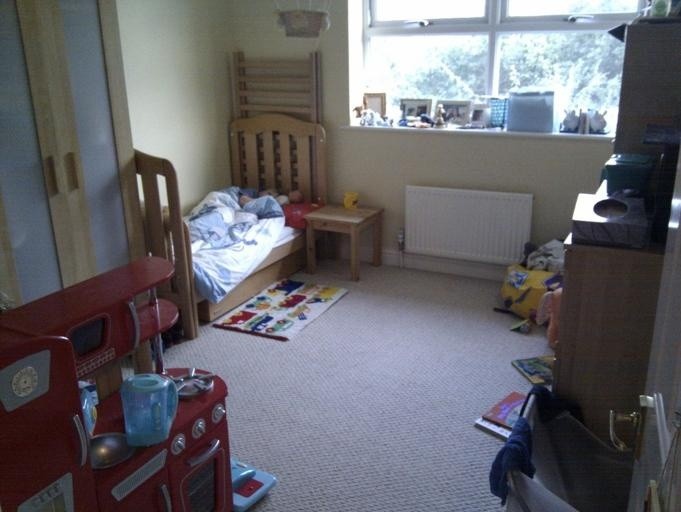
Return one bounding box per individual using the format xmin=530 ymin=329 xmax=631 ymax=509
xmin=403 ymin=183 xmax=537 ymax=266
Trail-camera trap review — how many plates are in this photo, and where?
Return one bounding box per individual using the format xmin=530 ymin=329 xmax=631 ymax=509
xmin=88 ymin=433 xmax=136 ymax=470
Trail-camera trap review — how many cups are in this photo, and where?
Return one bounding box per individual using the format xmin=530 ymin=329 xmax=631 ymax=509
xmin=651 ymin=0 xmax=672 ymax=16
xmin=344 ymin=192 xmax=359 ymax=210
xmin=121 ymin=372 xmax=180 ymax=448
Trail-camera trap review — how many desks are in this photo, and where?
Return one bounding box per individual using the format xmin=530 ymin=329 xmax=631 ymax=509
xmin=303 ymin=205 xmax=387 ymax=281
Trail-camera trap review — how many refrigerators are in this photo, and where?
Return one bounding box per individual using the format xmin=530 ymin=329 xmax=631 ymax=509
xmin=1 ymin=330 xmax=100 ymax=512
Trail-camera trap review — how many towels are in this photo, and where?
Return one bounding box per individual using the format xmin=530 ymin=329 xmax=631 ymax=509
xmin=486 ymin=415 xmax=537 ymax=507
xmin=515 ymin=381 xmax=588 ymax=426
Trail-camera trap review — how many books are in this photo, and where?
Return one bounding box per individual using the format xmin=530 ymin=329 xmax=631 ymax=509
xmin=474 ymin=417 xmax=511 ymax=440
xmin=483 ymin=390 xmax=528 ymax=430
xmin=511 ymin=352 xmax=555 ymax=385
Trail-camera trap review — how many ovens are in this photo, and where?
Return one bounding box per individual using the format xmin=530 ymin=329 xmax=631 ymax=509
xmin=168 ymin=423 xmax=235 ymax=511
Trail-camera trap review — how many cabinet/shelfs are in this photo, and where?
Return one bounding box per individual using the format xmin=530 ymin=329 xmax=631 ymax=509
xmin=0 ymin=2 xmax=153 ymax=404
xmin=611 ymin=18 xmax=681 ymax=213
xmin=552 ymin=231 xmax=665 ymax=446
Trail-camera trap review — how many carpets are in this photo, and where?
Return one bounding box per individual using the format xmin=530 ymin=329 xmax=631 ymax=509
xmin=212 ymin=276 xmax=350 ymax=342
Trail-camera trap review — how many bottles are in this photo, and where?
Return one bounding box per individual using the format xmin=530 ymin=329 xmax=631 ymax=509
xmin=561 ymin=107 xmax=609 ymax=135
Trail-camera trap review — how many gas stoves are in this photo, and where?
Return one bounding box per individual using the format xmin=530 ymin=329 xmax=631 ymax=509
xmin=154 ymin=367 xmax=229 ymax=459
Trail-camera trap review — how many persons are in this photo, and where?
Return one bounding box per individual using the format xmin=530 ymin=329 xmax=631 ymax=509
xmin=238 ymin=188 xmax=304 ymax=210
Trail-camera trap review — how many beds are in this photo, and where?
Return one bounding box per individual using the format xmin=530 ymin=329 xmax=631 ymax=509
xmin=133 ymin=112 xmax=333 ymax=341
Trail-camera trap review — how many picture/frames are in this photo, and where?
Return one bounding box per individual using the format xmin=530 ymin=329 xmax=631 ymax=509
xmin=400 ymin=96 xmax=433 ymax=118
xmin=360 ymin=92 xmax=387 ymax=117
xmin=437 ymin=99 xmax=472 ymax=127
